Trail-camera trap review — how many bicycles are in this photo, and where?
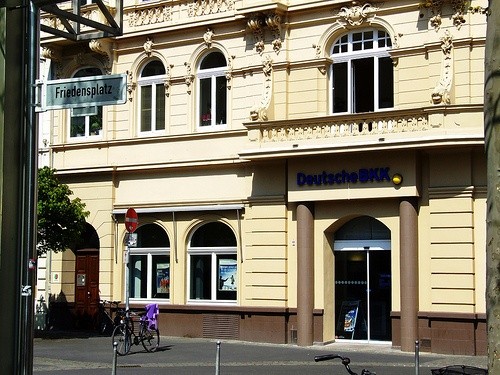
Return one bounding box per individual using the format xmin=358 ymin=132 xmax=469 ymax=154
xmin=112 ymin=309 xmax=161 ymax=356
xmin=96 ymin=299 xmax=133 ymax=336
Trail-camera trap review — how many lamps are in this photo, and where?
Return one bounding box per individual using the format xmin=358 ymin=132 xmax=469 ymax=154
xmin=392 ymin=173 xmax=404 ymax=185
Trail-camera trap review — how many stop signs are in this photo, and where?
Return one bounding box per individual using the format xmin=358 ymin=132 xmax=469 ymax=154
xmin=124 ymin=207 xmax=138 ymax=234
